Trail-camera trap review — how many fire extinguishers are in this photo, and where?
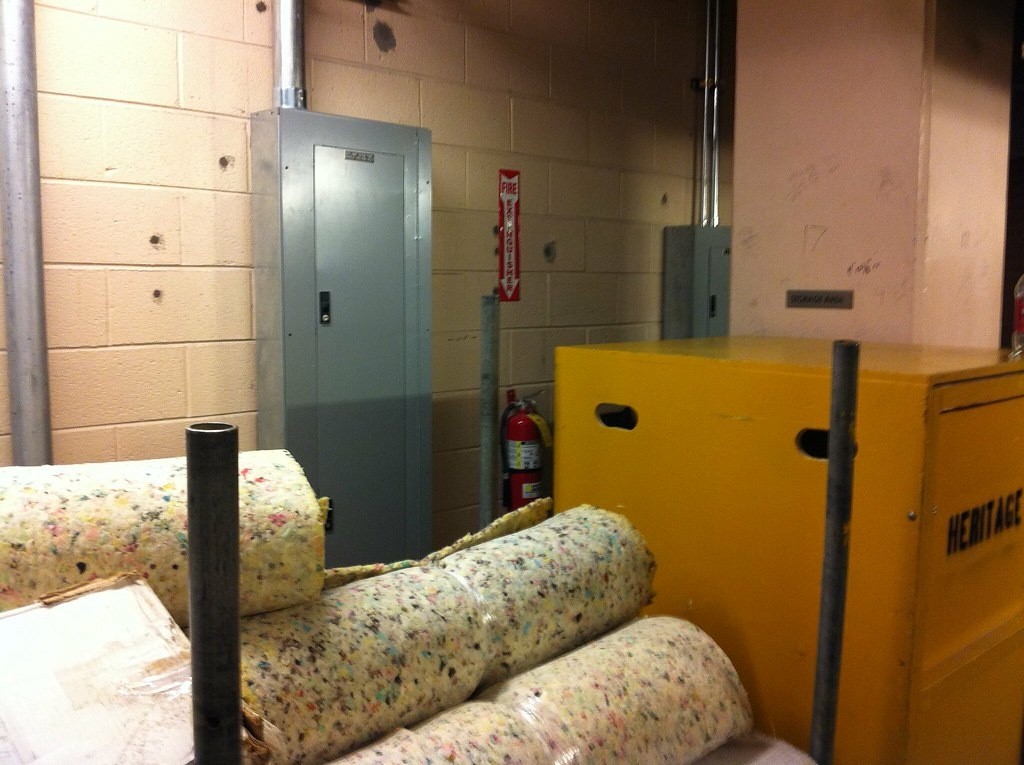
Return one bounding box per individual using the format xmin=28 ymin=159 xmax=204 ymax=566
xmin=499 ymin=389 xmax=548 ymax=512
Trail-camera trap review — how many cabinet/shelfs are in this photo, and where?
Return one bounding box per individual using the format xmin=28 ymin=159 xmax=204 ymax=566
xmin=554 ymin=338 xmax=1024 ymax=765
xmin=250 ymin=106 xmax=433 ymax=569
